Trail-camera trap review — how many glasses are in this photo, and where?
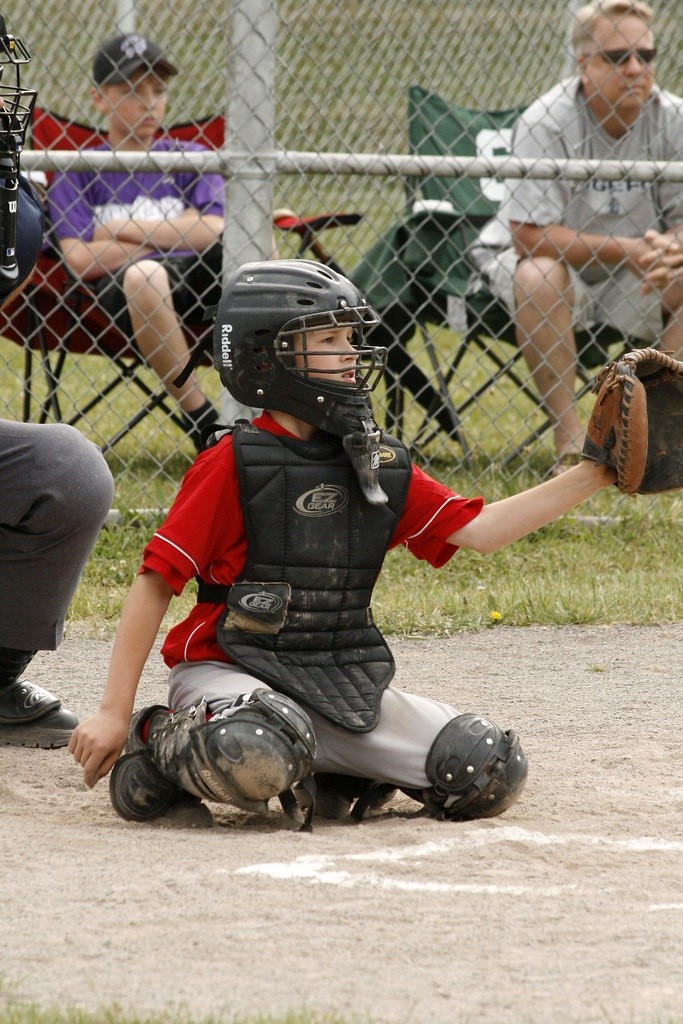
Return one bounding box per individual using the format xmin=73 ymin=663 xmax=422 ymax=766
xmin=583 ymin=46 xmax=657 ymax=66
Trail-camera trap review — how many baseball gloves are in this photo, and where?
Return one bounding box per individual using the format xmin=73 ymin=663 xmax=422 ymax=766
xmin=579 ymin=347 xmax=682 ymax=497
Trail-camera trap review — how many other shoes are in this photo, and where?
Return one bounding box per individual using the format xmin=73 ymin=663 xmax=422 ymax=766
xmin=124 ymin=704 xmax=213 ymax=830
xmin=302 ymin=774 xmax=369 ymax=818
xmin=553 ymin=452 xmax=579 ymax=475
xmin=0 ymin=678 xmax=80 ymax=749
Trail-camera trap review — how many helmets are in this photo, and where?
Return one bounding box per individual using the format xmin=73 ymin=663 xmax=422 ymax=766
xmin=212 ymin=261 xmax=388 ymax=505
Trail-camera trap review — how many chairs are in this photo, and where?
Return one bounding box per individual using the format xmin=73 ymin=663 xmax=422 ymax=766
xmin=403 ymin=85 xmax=659 ymax=473
xmin=9 ymin=103 xmax=363 ymax=452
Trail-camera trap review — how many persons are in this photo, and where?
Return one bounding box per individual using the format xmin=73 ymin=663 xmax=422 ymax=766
xmin=472 ymin=0 xmax=683 ymax=478
xmin=65 ymin=258 xmax=683 ymax=832
xmin=50 ymin=31 xmax=226 ymax=456
xmin=0 ymin=11 xmax=114 ymax=749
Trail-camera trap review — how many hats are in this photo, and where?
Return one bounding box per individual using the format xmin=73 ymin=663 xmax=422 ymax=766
xmin=93 ymin=35 xmax=175 ymax=84
